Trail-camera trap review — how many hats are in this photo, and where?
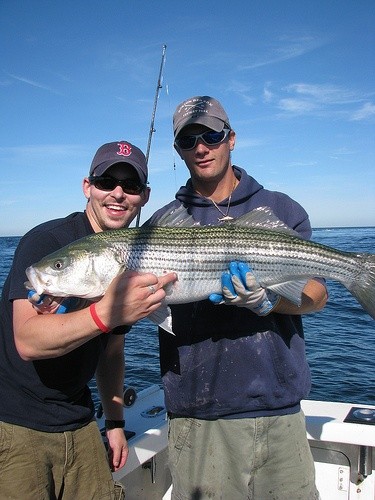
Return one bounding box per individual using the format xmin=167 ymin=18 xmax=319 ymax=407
xmin=172 ymin=96 xmax=231 ymax=141
xmin=90 ymin=141 xmax=148 ymax=187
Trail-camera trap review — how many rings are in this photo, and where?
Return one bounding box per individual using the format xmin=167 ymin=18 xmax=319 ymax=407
xmin=148 ymin=285 xmax=155 ymax=293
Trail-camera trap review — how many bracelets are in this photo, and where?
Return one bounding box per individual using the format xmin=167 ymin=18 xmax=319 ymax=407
xmin=90 ymin=303 xmax=111 ymax=333
xmin=105 ymin=420 xmax=125 ymax=428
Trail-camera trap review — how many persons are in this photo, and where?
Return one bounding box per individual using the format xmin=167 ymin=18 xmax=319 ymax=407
xmin=0 ymin=141 xmax=177 ymax=500
xmin=140 ymin=96 xmax=330 ymax=500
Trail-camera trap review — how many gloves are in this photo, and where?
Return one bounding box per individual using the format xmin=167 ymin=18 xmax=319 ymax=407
xmin=209 ymin=261 xmax=281 ymax=317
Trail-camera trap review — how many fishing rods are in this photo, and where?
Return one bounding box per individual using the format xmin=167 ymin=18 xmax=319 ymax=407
xmin=97 ymin=43 xmax=167 ymax=419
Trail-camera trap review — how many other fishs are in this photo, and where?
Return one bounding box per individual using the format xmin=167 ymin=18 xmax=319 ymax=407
xmin=24 ymin=196 xmax=375 ymax=337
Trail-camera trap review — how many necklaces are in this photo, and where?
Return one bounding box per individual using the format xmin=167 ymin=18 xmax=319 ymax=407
xmin=194 ymin=182 xmax=235 ymax=217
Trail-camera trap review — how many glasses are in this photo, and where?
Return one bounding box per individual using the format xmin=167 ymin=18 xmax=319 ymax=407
xmin=89 ymin=175 xmax=142 ymax=195
xmin=174 ymin=129 xmax=231 ymax=151
